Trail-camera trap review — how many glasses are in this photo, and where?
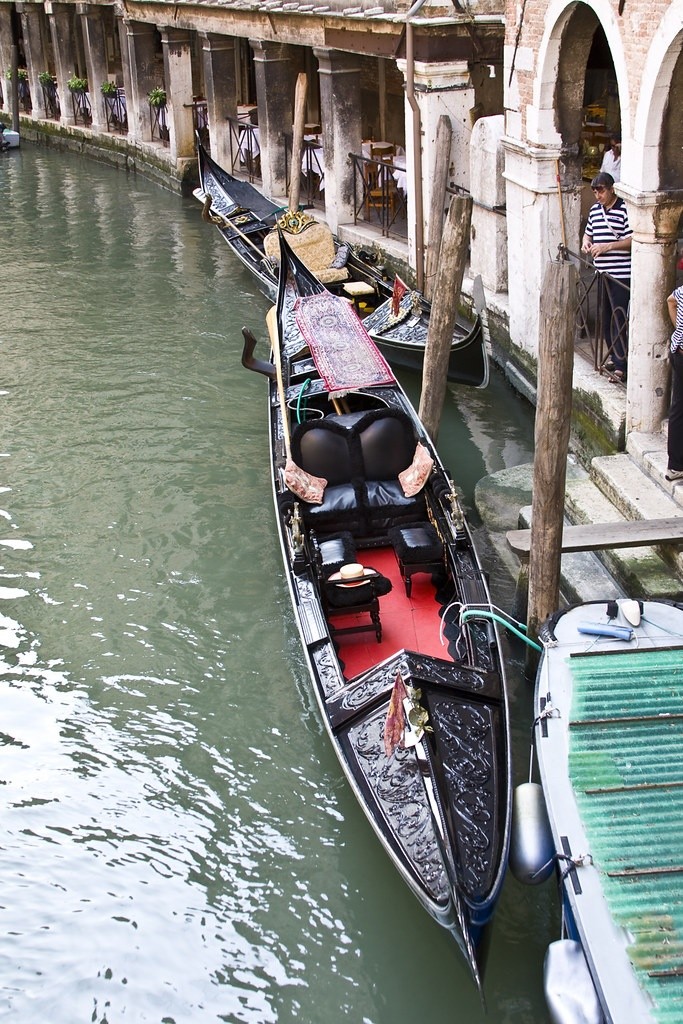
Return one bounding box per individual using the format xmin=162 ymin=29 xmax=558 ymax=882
xmin=591 ymin=185 xmax=608 ymax=192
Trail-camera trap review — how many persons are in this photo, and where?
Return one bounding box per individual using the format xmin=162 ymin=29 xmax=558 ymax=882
xmin=580 ymin=172 xmax=633 ymax=383
xmin=664 ymin=285 xmax=683 ymax=481
xmin=598 ymin=133 xmax=622 ymax=183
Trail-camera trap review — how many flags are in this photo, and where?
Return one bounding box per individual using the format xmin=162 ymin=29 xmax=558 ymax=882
xmin=390 ymin=277 xmax=407 ymax=317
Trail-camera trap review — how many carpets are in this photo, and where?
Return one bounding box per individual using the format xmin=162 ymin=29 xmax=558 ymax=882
xmin=291 ymin=293 xmax=397 ymax=401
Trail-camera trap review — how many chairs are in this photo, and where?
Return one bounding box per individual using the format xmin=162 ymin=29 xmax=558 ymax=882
xmin=265 ymin=211 xmax=352 ymax=305
xmin=364 ymin=155 xmax=405 ymax=224
xmin=236 ymin=100 xmax=257 ymax=106
xmin=370 ymin=141 xmax=407 ymax=219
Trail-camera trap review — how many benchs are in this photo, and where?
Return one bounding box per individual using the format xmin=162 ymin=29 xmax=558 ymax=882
xmin=308 ymin=529 xmax=382 ymax=646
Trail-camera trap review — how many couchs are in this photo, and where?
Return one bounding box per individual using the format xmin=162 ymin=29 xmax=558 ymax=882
xmin=290 ymin=409 xmax=429 ymax=547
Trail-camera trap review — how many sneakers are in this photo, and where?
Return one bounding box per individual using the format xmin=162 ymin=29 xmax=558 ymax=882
xmin=664 ymin=467 xmax=682 ymax=481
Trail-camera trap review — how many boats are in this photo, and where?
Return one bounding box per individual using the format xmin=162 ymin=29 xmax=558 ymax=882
xmin=238 ymin=222 xmax=510 ymax=1021
xmin=508 ymin=600 xmax=682 ymax=1024
xmin=192 ymin=128 xmax=492 ymax=391
xmin=0 ymin=121 xmax=21 ymax=151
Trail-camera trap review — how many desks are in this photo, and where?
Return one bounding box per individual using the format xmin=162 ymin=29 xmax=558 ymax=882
xmin=303 ymin=133 xmax=323 ymax=147
xmin=239 ymin=129 xmax=260 ymax=178
xmin=303 ymin=140 xmax=405 ymax=210
xmin=206 ymin=104 xmax=258 ymax=127
xmin=376 ymin=156 xmax=407 ymax=199
xmin=291 ymin=122 xmax=320 ymax=132
xmin=158 ymin=100 xmax=208 ymax=143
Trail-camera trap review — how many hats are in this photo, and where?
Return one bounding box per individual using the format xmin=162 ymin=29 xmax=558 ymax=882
xmin=327 ymin=563 xmax=377 ymax=588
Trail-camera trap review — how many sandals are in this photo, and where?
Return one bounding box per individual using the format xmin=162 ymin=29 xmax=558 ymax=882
xmin=608 ymin=370 xmax=625 ymax=383
xmin=602 ymin=362 xmax=623 ymax=371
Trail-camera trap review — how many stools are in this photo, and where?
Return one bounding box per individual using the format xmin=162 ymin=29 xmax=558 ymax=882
xmin=387 ymin=522 xmax=443 ymax=597
xmin=342 ymin=282 xmax=376 ymax=320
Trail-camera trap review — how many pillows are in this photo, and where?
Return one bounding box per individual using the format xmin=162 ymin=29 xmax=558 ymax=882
xmin=282 ymin=458 xmax=328 ymax=505
xmin=398 ymin=441 xmax=435 ymax=498
xmin=330 ymin=246 xmax=350 ymax=269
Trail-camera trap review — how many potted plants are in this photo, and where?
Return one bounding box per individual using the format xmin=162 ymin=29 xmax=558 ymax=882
xmin=37 ymin=71 xmax=55 ymax=89
xmin=67 ymin=75 xmax=87 ymax=94
xmin=5 ymin=69 xmax=27 ymax=83
xmin=147 ymin=88 xmax=167 ymax=108
xmin=99 ymin=81 xmax=119 ymax=99
